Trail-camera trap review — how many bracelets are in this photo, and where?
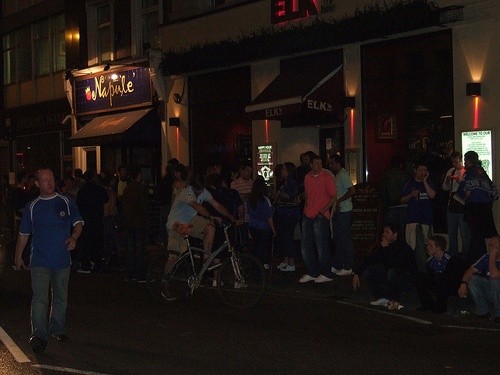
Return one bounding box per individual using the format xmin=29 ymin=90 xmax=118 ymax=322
xmin=461 ymin=281 xmax=467 ymax=285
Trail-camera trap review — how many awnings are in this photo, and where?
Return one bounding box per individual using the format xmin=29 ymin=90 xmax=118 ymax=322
xmin=68 ymin=106 xmax=160 ymax=147
xmin=245 ymin=63 xmax=342 ymax=120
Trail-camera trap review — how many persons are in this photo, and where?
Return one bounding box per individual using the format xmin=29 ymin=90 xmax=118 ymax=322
xmin=0 ymin=166 xmax=84 ymax=351
xmin=352 ymin=152 xmax=500 ymax=323
xmin=54 ymin=151 xmax=355 ymax=300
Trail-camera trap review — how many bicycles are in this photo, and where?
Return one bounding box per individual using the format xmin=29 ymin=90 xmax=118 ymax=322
xmin=157 ymin=218 xmax=266 ymax=310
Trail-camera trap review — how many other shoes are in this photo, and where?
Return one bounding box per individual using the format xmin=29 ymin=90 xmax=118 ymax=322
xmin=29 ymin=336 xmax=46 ymax=354
xmin=202 ymin=257 xmax=221 ymax=267
xmin=77 ymin=267 xmax=91 ymax=274
xmin=276 ymin=262 xmax=288 ymax=270
xmin=370 ymin=298 xmax=389 ymax=307
xmin=298 ymin=273 xmax=314 ymax=283
xmin=335 ymin=268 xmax=353 ymax=276
xmin=52 ymin=334 xmax=69 ymax=344
xmin=279 ymin=264 xmax=296 ymax=272
xmin=160 ymin=288 xmax=177 ymax=301
xmin=314 ymin=274 xmax=334 ymax=283
